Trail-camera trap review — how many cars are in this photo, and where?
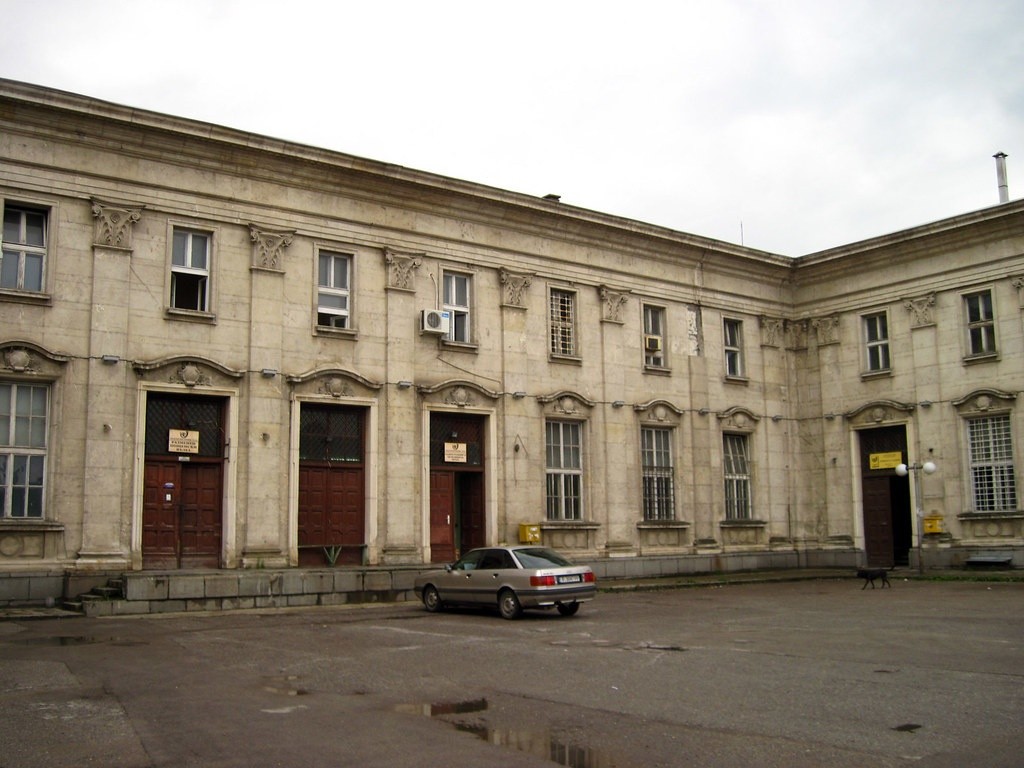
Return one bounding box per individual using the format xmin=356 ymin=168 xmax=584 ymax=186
xmin=414 ymin=544 xmax=597 ymax=620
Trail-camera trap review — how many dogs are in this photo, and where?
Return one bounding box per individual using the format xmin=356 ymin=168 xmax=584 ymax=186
xmin=856 ymin=566 xmax=895 ymax=590
